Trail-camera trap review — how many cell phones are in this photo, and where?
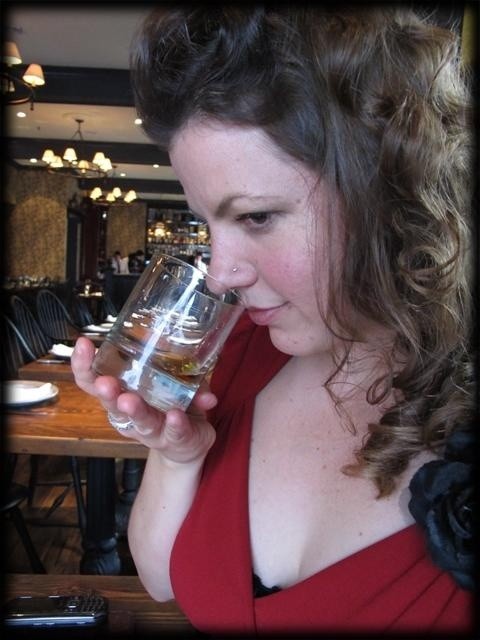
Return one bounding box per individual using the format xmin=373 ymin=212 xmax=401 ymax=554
xmin=0 ymin=595 xmax=110 ymax=629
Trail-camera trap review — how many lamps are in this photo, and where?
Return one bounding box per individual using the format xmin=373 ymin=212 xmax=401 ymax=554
xmin=2 ymin=27 xmax=46 ymax=111
xmin=90 ymin=165 xmax=137 ymax=203
xmin=42 ymin=118 xmax=113 ymax=173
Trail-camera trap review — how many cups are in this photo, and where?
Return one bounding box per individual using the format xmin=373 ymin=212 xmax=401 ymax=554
xmin=90 ymin=250 xmax=246 ymax=417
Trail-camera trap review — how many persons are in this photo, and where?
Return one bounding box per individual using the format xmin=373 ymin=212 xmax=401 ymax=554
xmin=70 ymin=2 xmax=478 ymax=639
xmin=194 ymin=252 xmax=208 ymax=275
xmin=129 ymin=250 xmax=144 ymax=268
xmin=113 ymin=251 xmax=130 ymax=273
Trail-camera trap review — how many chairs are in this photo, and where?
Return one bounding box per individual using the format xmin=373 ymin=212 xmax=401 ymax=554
xmin=0 ymin=290 xmax=95 ymax=575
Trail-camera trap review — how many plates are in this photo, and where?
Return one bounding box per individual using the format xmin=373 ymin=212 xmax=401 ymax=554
xmin=48 ymin=345 xmax=100 ymax=358
xmin=2 ymin=380 xmax=60 ymax=407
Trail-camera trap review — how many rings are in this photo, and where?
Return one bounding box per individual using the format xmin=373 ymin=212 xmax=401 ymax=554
xmin=107 ymin=413 xmax=134 ymax=432
